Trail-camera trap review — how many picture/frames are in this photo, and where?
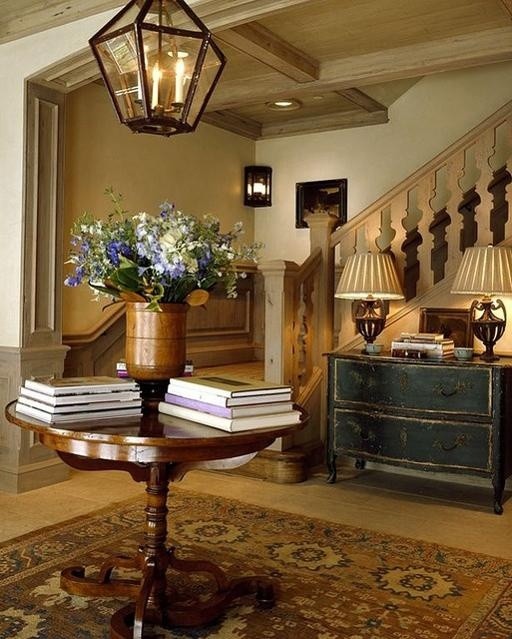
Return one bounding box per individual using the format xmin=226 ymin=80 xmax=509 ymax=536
xmin=243 ymin=165 xmax=272 ymax=207
xmin=295 ymin=177 xmax=347 ymax=230
xmin=418 ymin=306 xmax=475 ymax=350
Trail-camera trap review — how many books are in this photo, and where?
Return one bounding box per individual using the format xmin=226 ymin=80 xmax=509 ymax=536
xmin=158 ymin=374 xmax=303 ymax=432
xmin=390 ymin=331 xmax=456 ymax=358
xmin=14 ymin=375 xmax=144 ymax=424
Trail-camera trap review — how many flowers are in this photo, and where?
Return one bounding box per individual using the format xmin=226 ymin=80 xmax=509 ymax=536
xmin=63 ymin=183 xmax=265 ymax=311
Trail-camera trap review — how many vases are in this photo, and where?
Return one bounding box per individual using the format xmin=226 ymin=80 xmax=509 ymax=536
xmin=124 ymin=302 xmax=191 ymax=411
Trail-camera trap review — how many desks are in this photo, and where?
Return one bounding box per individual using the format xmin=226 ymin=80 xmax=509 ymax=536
xmin=4 ymin=400 xmax=311 ymax=639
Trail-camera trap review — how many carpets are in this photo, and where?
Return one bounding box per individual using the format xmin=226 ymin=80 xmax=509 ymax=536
xmin=0 ymin=484 xmax=512 ymax=639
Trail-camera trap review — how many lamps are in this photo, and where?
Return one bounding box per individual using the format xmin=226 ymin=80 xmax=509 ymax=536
xmin=88 ymin=0 xmax=227 ymax=138
xmin=450 ymin=243 xmax=512 ymax=362
xmin=333 ymin=250 xmax=406 ymax=356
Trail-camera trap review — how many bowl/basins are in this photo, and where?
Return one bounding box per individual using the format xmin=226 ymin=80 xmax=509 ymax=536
xmin=452 ymin=348 xmax=474 ymax=360
xmin=364 ymin=343 xmax=384 ymax=354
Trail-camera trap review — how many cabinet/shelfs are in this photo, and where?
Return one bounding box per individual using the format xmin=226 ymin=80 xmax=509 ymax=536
xmin=321 ymin=351 xmax=512 ymax=516
